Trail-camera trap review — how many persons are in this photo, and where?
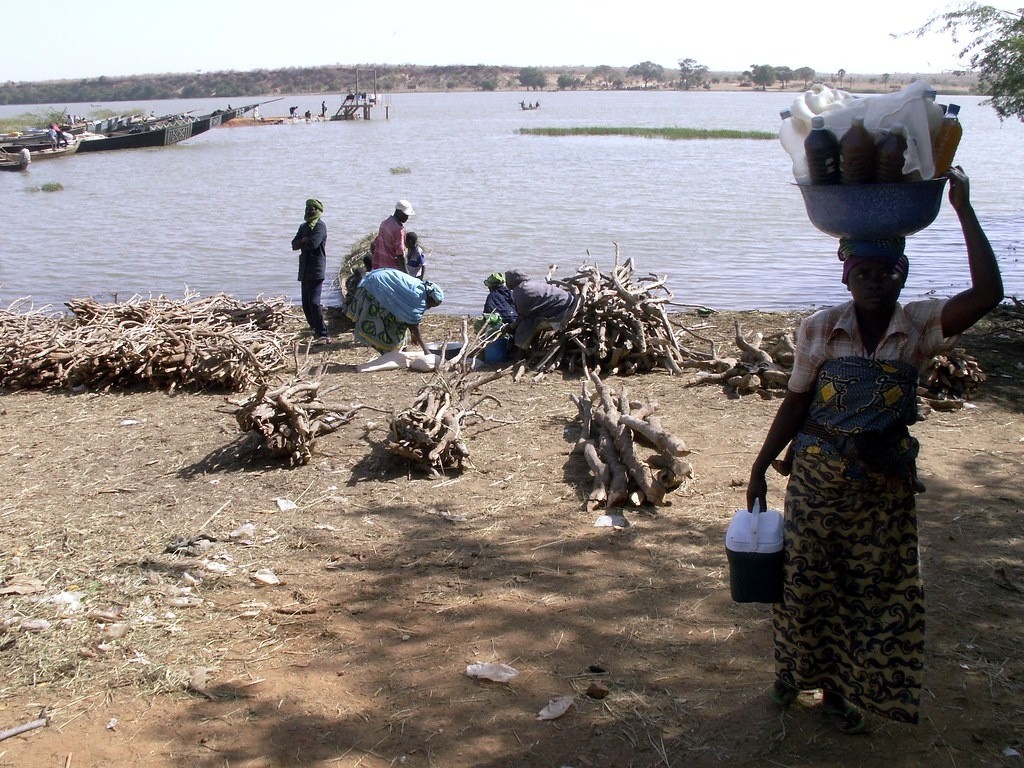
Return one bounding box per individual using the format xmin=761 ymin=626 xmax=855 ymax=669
xmin=469 ymin=273 xmax=518 ymax=359
xmin=49 ymin=122 xmax=69 ymax=148
xmin=305 ymin=111 xmax=311 ymax=119
xmin=289 ymin=106 xmax=298 ymax=118
xmin=519 ymin=101 xmax=525 ymax=107
xmin=18 ymin=145 xmax=31 ymax=162
xmin=48 ymin=129 xmax=58 ymax=151
xmin=404 ymin=232 xmax=426 ymax=280
xmin=253 ymin=107 xmax=259 ymax=121
xmin=321 ymin=101 xmax=326 ymax=117
xmin=346 ymin=268 xmax=443 ymax=357
xmin=747 ymin=164 xmax=1005 ymax=736
xmin=496 ymin=269 xmax=575 ymax=367
xmin=529 ymin=103 xmax=532 ymax=108
xmin=536 ymin=101 xmax=539 ymax=107
xmin=373 ymin=199 xmax=415 ymax=275
xmin=346 ymin=91 xmax=366 ymax=99
xmin=66 ymin=114 xmax=74 ymax=125
xmin=292 ymin=199 xmax=329 ymax=344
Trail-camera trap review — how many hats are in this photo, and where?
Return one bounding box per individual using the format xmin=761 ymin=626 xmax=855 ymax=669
xmin=304 ymin=198 xmax=324 ymax=229
xmin=395 ymin=199 xmax=416 ymax=216
xmin=483 ymin=272 xmax=505 ymax=288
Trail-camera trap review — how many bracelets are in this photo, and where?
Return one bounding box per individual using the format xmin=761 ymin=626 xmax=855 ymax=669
xmin=411 ymin=334 xmax=415 ymax=336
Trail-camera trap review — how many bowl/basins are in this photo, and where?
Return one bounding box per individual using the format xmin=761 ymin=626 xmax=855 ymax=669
xmin=789 ymin=169 xmax=949 ymax=239
xmin=426 ymin=340 xmax=462 ymax=359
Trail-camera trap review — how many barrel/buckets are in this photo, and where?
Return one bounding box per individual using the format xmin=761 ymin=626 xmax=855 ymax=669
xmin=483 ymin=337 xmax=508 ymax=362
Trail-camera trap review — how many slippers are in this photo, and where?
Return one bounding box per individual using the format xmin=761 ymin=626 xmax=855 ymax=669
xmin=770 ymin=682 xmax=800 ymax=705
xmin=821 ymin=699 xmax=866 ymax=732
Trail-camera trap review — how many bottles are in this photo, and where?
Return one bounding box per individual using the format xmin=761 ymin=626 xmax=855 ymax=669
xmin=777 ymin=84 xmax=964 ymax=182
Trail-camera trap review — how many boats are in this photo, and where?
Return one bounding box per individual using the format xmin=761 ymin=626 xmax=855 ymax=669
xmin=0 ymin=97 xmax=284 ymax=170
xmin=522 ymin=107 xmax=539 ymax=110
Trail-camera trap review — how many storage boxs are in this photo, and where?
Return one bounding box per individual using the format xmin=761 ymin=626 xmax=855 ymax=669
xmin=725 ymin=497 xmax=785 ymax=604
